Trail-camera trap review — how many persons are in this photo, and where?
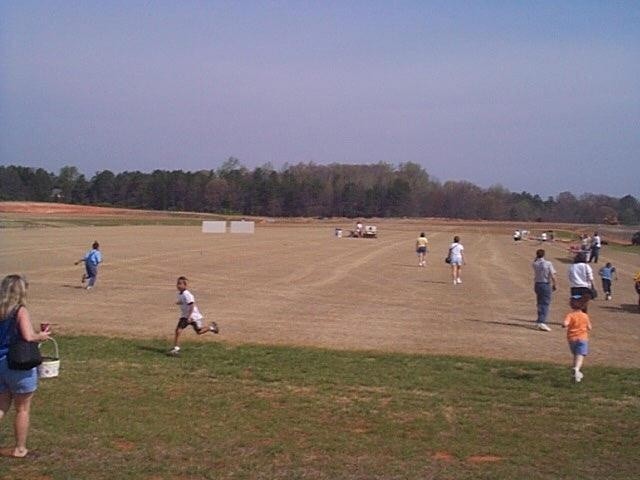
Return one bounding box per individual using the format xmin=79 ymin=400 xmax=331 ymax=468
xmin=415 ymin=233 xmax=428 ymax=267
xmin=75 ymin=242 xmax=104 ymax=289
xmin=168 ymin=277 xmax=218 ymax=354
xmin=514 ymin=229 xmax=640 ymax=383
xmin=357 ymin=222 xmax=363 ymax=236
xmin=446 ymin=236 xmax=466 ymax=286
xmin=0 ymin=275 xmax=51 ymax=459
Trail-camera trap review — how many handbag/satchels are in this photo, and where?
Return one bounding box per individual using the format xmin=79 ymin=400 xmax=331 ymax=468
xmin=446 ymin=257 xmax=450 ymax=263
xmin=8 ymin=304 xmax=42 ymax=370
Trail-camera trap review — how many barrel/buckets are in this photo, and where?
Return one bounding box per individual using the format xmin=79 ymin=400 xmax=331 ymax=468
xmin=335 ymin=229 xmax=343 ymax=238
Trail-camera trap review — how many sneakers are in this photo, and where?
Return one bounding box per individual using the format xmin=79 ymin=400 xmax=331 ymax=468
xmin=541 ymin=323 xmax=551 ymax=331
xmin=533 ymin=323 xmax=540 ymax=330
xmin=571 ymin=367 xmax=577 ymax=384
xmin=577 ymin=370 xmax=584 ymax=383
xmin=211 ymin=322 xmax=219 ymax=333
xmin=167 ymin=348 xmax=179 ymax=357
